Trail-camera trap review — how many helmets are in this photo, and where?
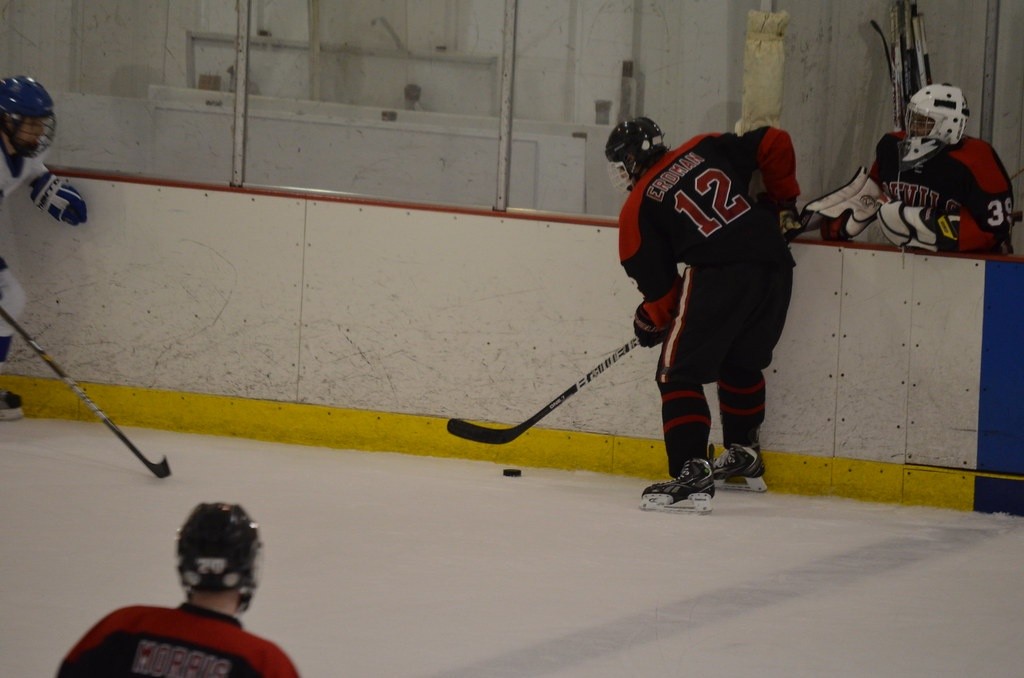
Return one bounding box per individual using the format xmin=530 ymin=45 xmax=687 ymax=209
xmin=604 ymin=117 xmax=663 ymax=191
xmin=902 ymin=83 xmax=969 ymax=161
xmin=176 ymin=502 xmax=262 ymax=613
xmin=0 ymin=76 xmax=56 ymax=157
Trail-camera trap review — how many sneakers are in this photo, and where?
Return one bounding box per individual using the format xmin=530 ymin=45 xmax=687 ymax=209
xmin=0 ymin=387 xmax=24 ymax=420
xmin=642 ymin=444 xmax=715 ymax=514
xmin=714 ymin=425 xmax=768 ymax=492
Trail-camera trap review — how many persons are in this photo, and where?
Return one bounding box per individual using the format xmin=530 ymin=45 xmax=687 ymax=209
xmin=55 ymin=503 xmax=303 ymax=677
xmin=604 ymin=115 xmax=802 ymax=516
xmin=0 ymin=75 xmax=87 ymax=421
xmin=822 ymin=83 xmax=1015 ymax=253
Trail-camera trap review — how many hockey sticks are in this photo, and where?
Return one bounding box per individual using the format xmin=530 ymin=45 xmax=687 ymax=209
xmin=870 ymin=1 xmax=935 ymax=131
xmin=447 ymin=335 xmax=639 ymax=444
xmin=0 ymin=303 xmax=170 ymax=477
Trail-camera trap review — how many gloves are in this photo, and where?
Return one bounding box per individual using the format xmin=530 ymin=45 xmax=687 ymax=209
xmin=31 ymin=172 xmax=87 ymax=225
xmin=779 ymin=202 xmax=804 ymax=241
xmin=633 ymin=302 xmax=669 ymax=349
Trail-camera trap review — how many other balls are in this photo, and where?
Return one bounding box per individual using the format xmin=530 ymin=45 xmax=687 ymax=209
xmin=502 ymin=469 xmax=522 ymax=477
